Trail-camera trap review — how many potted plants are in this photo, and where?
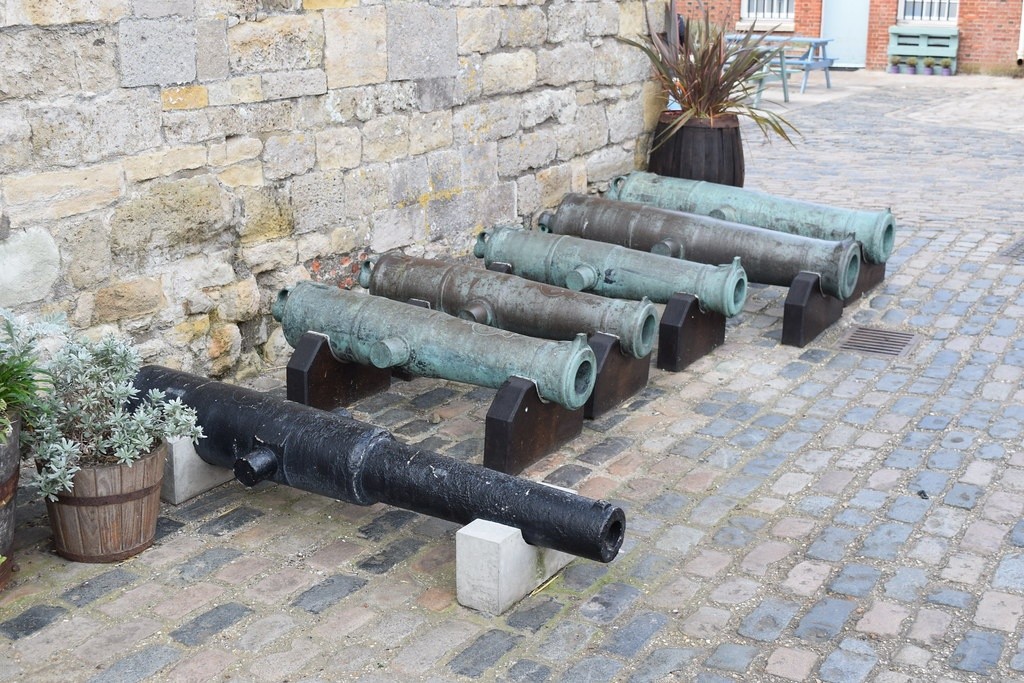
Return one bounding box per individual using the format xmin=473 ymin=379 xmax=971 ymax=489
xmin=941 ymin=59 xmax=951 ymax=76
xmin=34 ymin=335 xmax=206 ymax=558
xmin=889 ymin=56 xmax=900 ymax=73
xmin=619 ymin=0 xmax=800 ymax=187
xmin=923 ymin=57 xmax=934 ymax=75
xmin=906 ymin=58 xmax=918 ymax=74
xmin=0 ymin=339 xmax=56 ymax=555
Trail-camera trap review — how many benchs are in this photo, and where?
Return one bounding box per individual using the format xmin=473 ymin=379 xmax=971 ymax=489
xmin=729 ymin=56 xmax=838 ymax=84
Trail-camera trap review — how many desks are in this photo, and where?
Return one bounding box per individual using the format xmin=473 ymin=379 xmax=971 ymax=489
xmin=727 ymin=46 xmax=791 ymax=108
xmin=727 ymin=33 xmax=834 ymax=93
